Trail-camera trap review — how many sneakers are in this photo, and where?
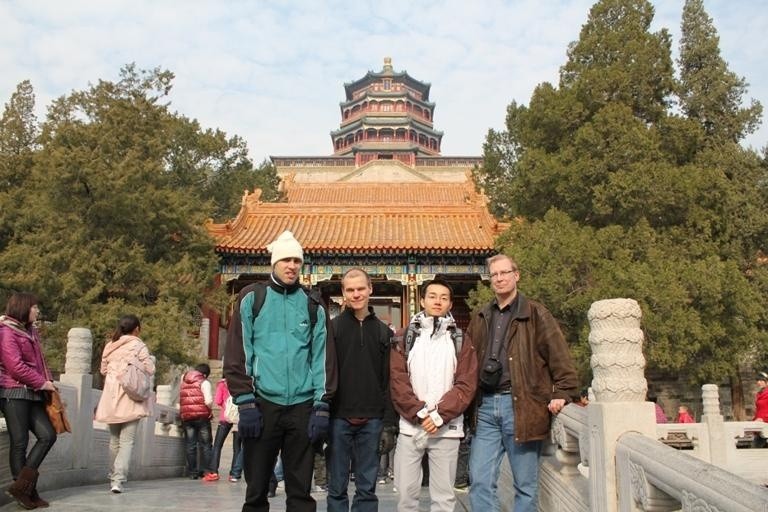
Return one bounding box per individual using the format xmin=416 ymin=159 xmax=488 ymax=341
xmin=111 ymin=482 xmax=124 ymax=493
xmin=189 ymin=471 xmax=238 ymax=482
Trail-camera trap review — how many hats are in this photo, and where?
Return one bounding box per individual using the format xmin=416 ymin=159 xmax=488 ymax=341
xmin=270 ymin=228 xmax=304 ymax=267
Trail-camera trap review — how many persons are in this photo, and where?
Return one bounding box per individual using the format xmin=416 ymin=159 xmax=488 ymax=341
xmin=464 ymin=253 xmax=579 ymax=512
xmin=646 ymin=391 xmax=665 ymax=424
xmin=748 ymin=367 xmax=767 ymax=449
xmin=0 ymin=292 xmax=59 ymax=510
xmin=388 ymin=275 xmax=475 ymax=512
xmin=676 ymin=405 xmax=694 ymax=423
xmin=262 ymin=442 xmax=472 ymax=497
xmin=574 ymin=391 xmax=589 ymax=403
xmin=92 ymin=314 xmax=155 ymax=494
xmin=325 ymin=267 xmax=397 ymax=512
xmin=178 ymin=363 xmax=213 ymax=479
xmin=202 ymin=371 xmax=247 ymax=482
xmin=222 ymin=229 xmax=340 ymax=512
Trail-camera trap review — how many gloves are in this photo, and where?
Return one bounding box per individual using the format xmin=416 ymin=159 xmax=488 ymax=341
xmin=380 ymin=431 xmax=395 ymax=455
xmin=238 ymin=403 xmax=263 ymax=438
xmin=308 ymin=404 xmax=330 ymax=448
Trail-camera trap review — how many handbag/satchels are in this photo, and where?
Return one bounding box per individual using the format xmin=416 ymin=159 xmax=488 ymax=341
xmin=45 ymin=388 xmax=71 ymax=434
xmin=225 ymin=396 xmax=240 ymax=424
xmin=117 ymin=357 xmax=151 ymax=401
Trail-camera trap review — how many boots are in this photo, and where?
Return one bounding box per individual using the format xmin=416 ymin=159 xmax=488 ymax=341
xmin=5 ymin=466 xmax=48 ymax=510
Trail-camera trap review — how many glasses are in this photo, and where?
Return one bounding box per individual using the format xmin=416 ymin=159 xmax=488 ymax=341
xmin=490 ymin=268 xmax=514 ymax=279
